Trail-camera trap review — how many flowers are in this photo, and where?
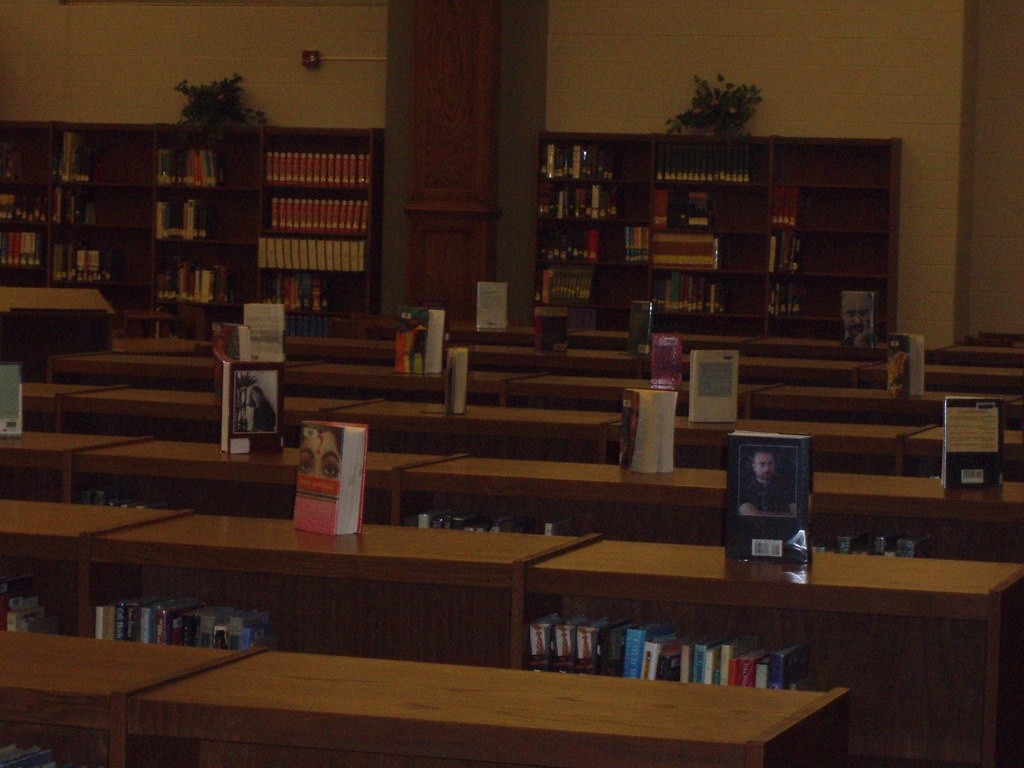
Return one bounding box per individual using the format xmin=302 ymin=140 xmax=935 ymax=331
xmin=169 ymin=68 xmax=268 ymax=126
xmin=667 ymin=64 xmax=771 ymax=139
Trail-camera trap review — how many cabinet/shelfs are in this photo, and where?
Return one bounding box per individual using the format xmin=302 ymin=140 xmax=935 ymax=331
xmin=0 ymin=118 xmax=1024 ymax=768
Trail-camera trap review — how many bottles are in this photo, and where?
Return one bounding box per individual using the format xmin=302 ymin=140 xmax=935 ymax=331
xmin=627 ymin=299 xmax=653 ymax=357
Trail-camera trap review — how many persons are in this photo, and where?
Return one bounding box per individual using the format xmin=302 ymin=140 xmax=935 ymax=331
xmin=739 ymin=451 xmax=795 ymax=516
xmin=842 ymin=295 xmax=871 ymax=348
xmin=299 ymin=423 xmax=342 ymax=477
xmin=248 ymin=386 xmax=276 ymax=432
xmin=620 ymin=398 xmax=639 ymax=467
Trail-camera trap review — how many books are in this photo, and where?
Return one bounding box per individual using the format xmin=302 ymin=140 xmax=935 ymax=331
xmin=394 ymin=307 xmax=444 ymax=374
xmin=95 ymin=594 xmax=273 ymax=650
xmin=529 ymin=609 xmax=809 ymax=692
xmin=292 ymin=419 xmax=369 ymax=536
xmin=533 ymin=141 xmax=805 ymax=314
xmin=443 ymin=348 xmax=469 ymax=415
xmin=535 ymin=306 xmax=569 ymax=355
xmin=811 ymin=530 xmax=932 ymax=558
xmin=399 ymin=507 xmax=560 ymax=536
xmin=80 ymin=486 xmax=152 ymax=510
xmin=727 ymin=430 xmax=812 ymax=563
xmin=0 ymin=741 xmax=57 ymax=768
xmin=0 ymin=364 xmax=24 ymax=439
xmin=0 ymin=132 xmax=371 ymax=399
xmin=0 ymin=576 xmax=46 ymax=633
xmin=619 ymin=292 xmax=927 ymax=473
xmin=476 ymin=282 xmax=509 ymax=329
xmin=941 ymin=397 xmax=1005 ymax=488
xmin=220 ymin=361 xmax=284 ymax=454
